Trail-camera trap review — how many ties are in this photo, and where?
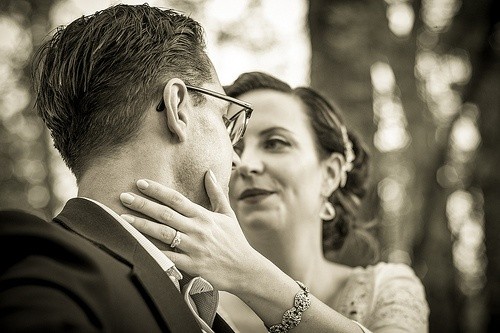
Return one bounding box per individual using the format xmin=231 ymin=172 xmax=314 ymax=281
xmin=177 ymin=271 xmax=235 ymax=333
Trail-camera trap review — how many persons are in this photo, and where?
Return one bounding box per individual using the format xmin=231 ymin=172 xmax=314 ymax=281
xmin=117 ymin=72 xmax=431 ymax=333
xmin=1 ymin=5 xmax=255 ymax=333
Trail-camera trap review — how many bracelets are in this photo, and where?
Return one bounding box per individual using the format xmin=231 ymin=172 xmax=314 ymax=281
xmin=262 ymin=279 xmax=311 ymax=333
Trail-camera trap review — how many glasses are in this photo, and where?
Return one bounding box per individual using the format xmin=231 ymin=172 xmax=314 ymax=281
xmin=157 ymin=82 xmax=255 ymax=149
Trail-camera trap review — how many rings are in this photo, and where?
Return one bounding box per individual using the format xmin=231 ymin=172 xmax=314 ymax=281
xmin=169 ymin=230 xmax=181 ymax=248
